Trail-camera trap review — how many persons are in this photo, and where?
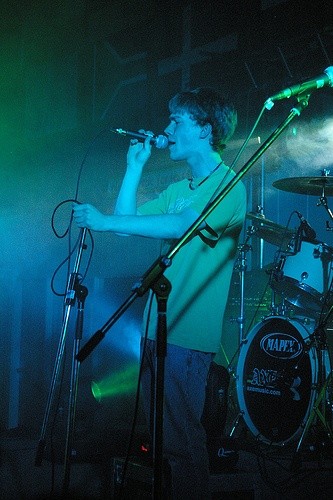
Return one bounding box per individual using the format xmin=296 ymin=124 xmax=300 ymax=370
xmin=72 ymin=86 xmax=247 ymax=500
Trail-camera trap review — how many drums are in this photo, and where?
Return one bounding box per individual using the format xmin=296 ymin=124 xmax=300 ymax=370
xmin=234 ymin=315 xmax=330 ymax=445
xmin=271 ymin=232 xmax=333 ymax=312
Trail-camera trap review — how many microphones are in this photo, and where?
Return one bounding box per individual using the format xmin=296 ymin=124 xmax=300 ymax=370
xmin=114 ymin=128 xmax=168 ymax=149
xmin=296 ymin=211 xmax=316 ymax=240
xmin=265 ymin=66 xmax=333 ymax=104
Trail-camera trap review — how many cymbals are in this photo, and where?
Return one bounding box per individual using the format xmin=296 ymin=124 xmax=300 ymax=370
xmin=271 ymin=168 xmax=333 ymax=197
